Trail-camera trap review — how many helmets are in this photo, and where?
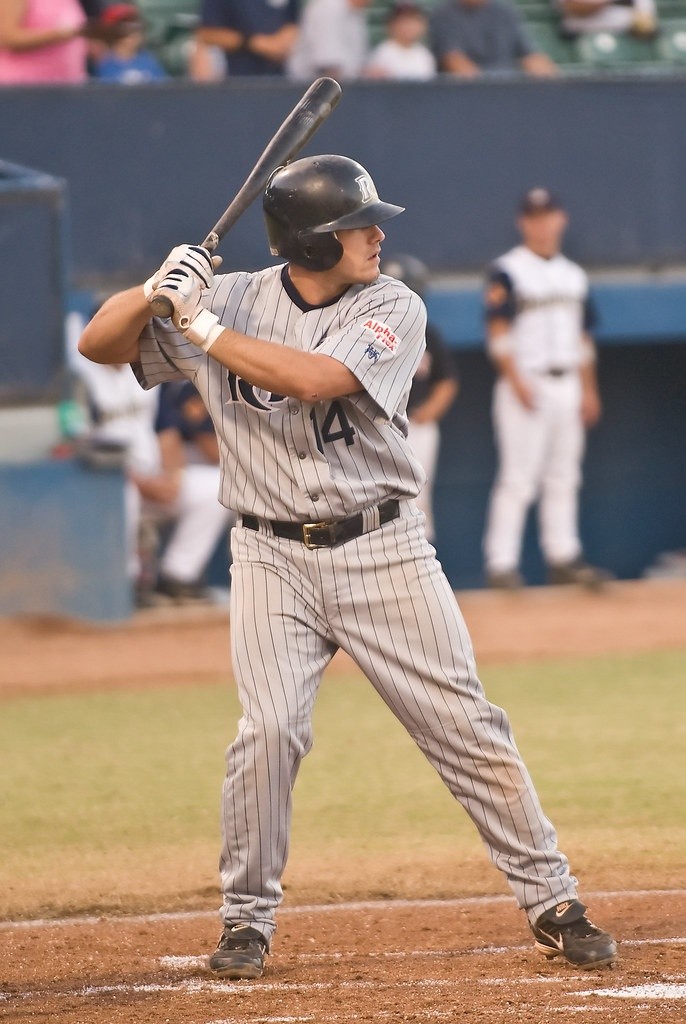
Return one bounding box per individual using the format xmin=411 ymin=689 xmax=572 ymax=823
xmin=262 ymin=154 xmax=405 ymax=271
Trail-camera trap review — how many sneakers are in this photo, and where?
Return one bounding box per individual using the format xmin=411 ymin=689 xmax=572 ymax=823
xmin=210 ymin=924 xmax=266 ymax=979
xmin=527 ymin=899 xmax=618 ymax=970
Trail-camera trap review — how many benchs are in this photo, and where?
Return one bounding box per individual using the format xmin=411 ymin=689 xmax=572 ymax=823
xmin=137 ymin=0 xmax=686 ymax=83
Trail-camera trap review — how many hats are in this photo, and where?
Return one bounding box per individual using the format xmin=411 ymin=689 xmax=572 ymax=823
xmin=518 ymin=185 xmax=562 ymax=217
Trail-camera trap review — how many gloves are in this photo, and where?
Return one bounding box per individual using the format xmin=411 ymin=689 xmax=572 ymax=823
xmin=146 ymin=259 xmax=225 ymax=353
xmin=143 ymin=244 xmax=216 ymax=299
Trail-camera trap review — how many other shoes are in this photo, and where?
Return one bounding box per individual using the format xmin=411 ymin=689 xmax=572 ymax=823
xmin=496 ymin=570 xmax=528 ymax=590
xmin=549 ymin=559 xmax=612 ymax=587
xmin=143 ymin=572 xmax=210 ymax=608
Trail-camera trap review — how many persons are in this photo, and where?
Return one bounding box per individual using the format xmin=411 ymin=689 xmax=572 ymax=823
xmin=0 ymin=0 xmax=686 ymax=609
xmin=78 ymin=154 xmax=623 ymax=973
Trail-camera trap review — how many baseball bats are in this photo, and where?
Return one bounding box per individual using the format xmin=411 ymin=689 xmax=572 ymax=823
xmin=148 ymin=77 xmax=345 ymax=321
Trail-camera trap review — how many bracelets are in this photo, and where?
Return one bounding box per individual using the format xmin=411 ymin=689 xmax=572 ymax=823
xmin=200 ymin=324 xmax=226 ymax=354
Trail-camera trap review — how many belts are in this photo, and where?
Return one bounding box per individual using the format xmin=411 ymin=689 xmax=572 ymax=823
xmin=242 ymin=499 xmax=399 ymax=548
